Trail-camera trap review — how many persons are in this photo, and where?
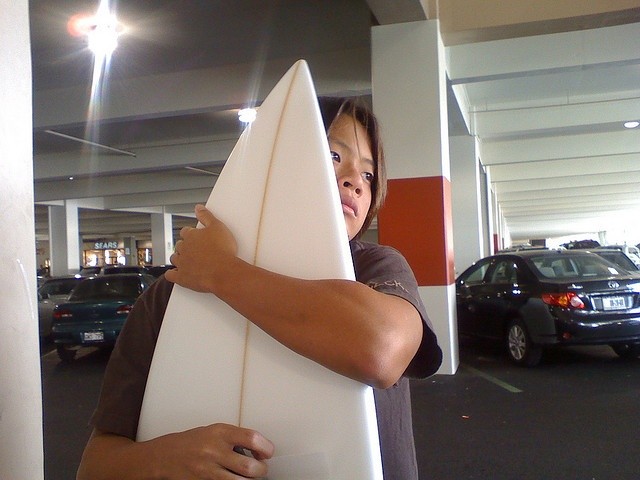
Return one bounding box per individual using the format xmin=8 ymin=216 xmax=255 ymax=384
xmin=75 ymin=96 xmax=442 ymax=480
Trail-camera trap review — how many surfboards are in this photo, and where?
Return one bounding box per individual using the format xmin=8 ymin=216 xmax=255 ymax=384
xmin=134 ymin=59 xmax=384 ymax=480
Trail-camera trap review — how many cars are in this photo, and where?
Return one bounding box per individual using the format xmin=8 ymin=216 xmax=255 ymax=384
xmin=606 ymin=241 xmax=633 ymax=248
xmin=51 ymin=273 xmax=158 ymax=361
xmin=37 ymin=274 xmax=95 ymax=346
xmin=455 ymin=250 xmax=640 ymax=368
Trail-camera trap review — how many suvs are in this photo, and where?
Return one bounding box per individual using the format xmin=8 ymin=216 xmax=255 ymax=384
xmin=562 ymin=237 xmax=605 ymax=249
